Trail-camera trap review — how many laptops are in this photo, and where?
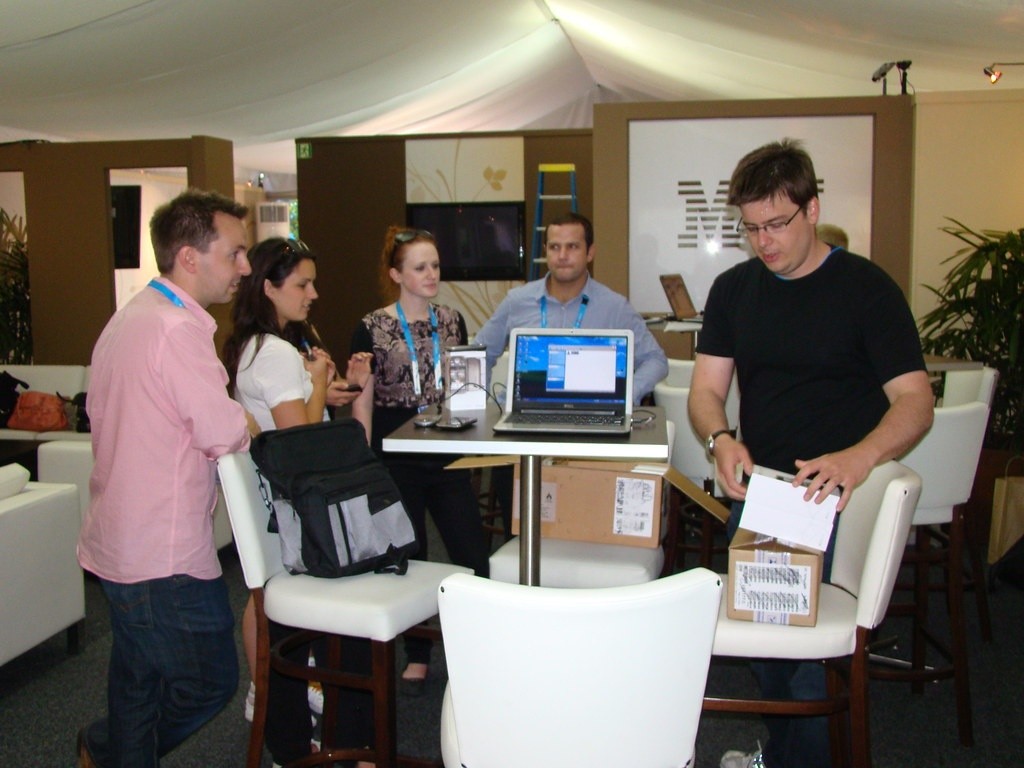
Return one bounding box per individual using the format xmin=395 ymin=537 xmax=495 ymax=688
xmin=660 ymin=274 xmax=704 ymax=323
xmin=492 ymin=327 xmax=635 ymax=435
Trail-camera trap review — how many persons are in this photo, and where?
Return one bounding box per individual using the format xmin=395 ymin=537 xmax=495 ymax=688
xmin=221 ymin=235 xmax=379 ymax=730
xmin=349 ymin=225 xmax=492 ymax=697
xmin=470 ymin=212 xmax=669 ymax=408
xmin=687 ymin=140 xmax=933 ymax=768
xmin=76 ymin=188 xmax=261 ymax=768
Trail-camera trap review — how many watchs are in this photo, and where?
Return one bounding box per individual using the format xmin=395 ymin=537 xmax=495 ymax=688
xmin=707 ymin=430 xmax=736 ymax=456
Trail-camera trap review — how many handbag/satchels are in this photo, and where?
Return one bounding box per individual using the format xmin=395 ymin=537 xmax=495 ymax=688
xmin=0 ymin=371 xmax=91 ymax=434
xmin=257 ymin=415 xmax=419 ymax=577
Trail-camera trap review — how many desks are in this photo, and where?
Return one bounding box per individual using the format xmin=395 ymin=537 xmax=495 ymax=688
xmin=382 ymin=408 xmax=669 ymax=590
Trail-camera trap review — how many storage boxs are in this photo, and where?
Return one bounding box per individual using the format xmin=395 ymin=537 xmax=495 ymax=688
xmin=734 ymin=465 xmax=843 ymax=551
xmin=440 ymin=459 xmax=726 ymax=550
xmin=729 ymin=531 xmax=824 ymax=626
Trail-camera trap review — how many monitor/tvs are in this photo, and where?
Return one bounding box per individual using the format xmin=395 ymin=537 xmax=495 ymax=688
xmin=402 ymin=200 xmax=525 ymax=280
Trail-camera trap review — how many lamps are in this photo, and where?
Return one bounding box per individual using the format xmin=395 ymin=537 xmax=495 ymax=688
xmin=983 ymin=61 xmax=1024 ymax=84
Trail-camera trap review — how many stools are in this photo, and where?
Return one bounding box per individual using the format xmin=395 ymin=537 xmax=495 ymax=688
xmin=207 ymin=335 xmax=995 ymax=767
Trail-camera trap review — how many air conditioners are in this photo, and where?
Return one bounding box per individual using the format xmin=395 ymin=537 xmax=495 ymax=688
xmin=255 ymin=200 xmax=291 ymax=243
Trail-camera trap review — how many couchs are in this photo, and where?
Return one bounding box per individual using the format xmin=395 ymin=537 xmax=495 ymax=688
xmin=1 ymin=366 xmax=239 ymax=660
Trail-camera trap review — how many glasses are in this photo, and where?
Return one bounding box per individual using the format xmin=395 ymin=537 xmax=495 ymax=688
xmin=735 ymin=205 xmax=803 ymax=237
xmin=274 ymin=238 xmax=311 ymax=273
xmin=389 ymin=227 xmax=435 ymax=268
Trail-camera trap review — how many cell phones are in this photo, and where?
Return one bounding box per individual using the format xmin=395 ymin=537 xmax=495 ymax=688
xmin=413 ymin=414 xmax=442 ymax=426
xmin=436 ymin=417 xmax=478 ymax=429
xmin=338 ymin=384 xmax=364 ymax=393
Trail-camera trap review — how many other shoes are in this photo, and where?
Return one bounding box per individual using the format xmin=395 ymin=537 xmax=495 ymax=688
xmin=244 ymin=683 xmax=318 ymax=726
xmin=304 ymin=680 xmax=325 ymax=713
xmin=720 ymin=741 xmax=764 ymax=767
xmin=399 ymin=662 xmax=429 ymax=695
xmin=74 ymin=729 xmax=93 ymax=767
